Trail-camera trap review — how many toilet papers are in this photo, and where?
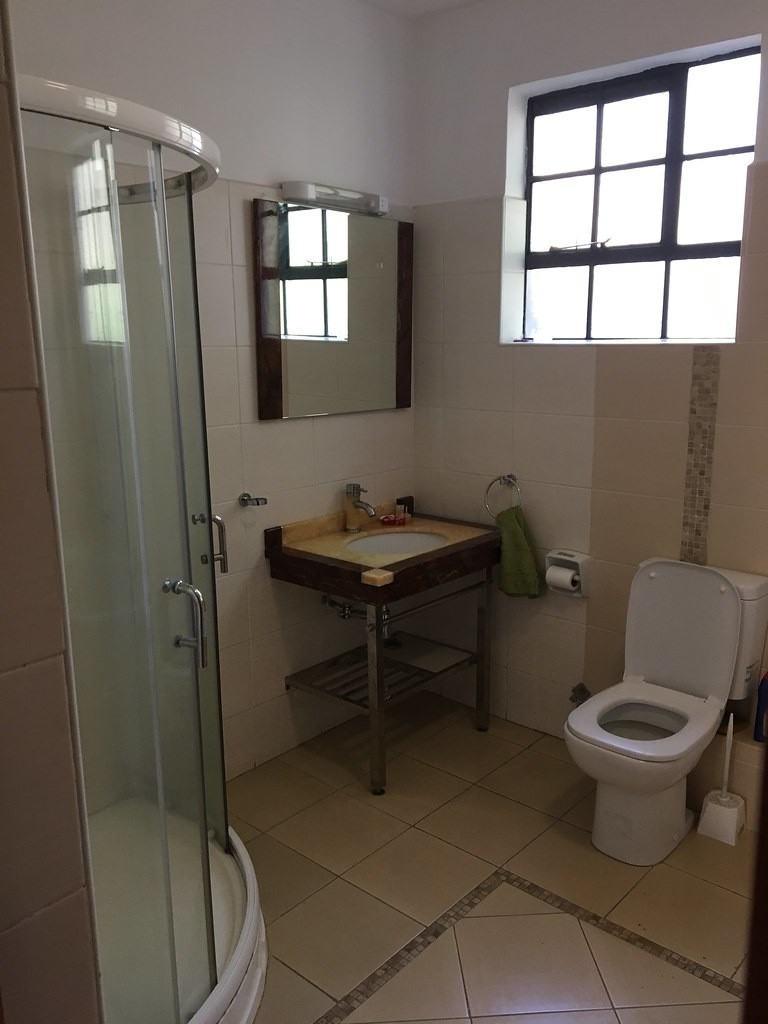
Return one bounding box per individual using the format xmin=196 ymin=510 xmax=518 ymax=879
xmin=546 ymin=565 xmax=580 ymax=591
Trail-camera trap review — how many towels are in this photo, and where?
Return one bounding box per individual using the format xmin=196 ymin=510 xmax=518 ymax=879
xmin=494 ymin=506 xmax=542 ymax=598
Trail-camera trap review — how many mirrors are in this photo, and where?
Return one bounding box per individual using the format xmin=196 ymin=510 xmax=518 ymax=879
xmin=253 ymin=198 xmax=415 ymax=421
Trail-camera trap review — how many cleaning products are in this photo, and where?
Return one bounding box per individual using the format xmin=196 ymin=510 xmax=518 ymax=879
xmin=754 ymin=670 xmax=768 ymax=742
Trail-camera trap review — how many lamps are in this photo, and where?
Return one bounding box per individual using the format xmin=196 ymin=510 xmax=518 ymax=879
xmin=282 ymin=180 xmax=389 ymax=217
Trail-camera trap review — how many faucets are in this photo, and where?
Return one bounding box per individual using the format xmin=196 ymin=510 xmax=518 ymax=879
xmin=344 ymin=483 xmax=376 ymax=533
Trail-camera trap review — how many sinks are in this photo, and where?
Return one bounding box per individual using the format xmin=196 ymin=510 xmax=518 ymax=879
xmin=345 ymin=530 xmax=450 ymax=554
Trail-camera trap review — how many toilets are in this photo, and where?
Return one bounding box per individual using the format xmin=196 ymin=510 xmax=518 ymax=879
xmin=562 ymin=556 xmax=768 ymax=867
